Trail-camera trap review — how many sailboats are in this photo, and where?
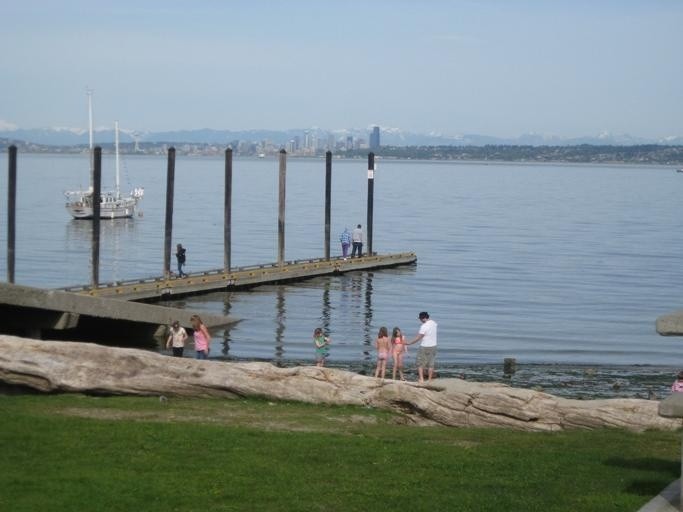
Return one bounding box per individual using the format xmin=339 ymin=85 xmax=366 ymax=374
xmin=62 ymin=90 xmax=147 ymax=221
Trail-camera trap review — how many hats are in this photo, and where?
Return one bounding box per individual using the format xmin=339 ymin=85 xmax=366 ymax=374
xmin=419 ymin=312 xmax=427 ymax=318
xmin=315 ymin=328 xmax=322 ymax=333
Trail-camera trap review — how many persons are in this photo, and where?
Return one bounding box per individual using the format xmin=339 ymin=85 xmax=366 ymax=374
xmin=165 ymin=323 xmax=188 ymax=358
xmin=374 ymin=327 xmax=409 ymax=381
xmin=339 ymin=225 xmax=363 ymax=260
xmin=400 ymin=312 xmax=439 ymax=383
xmin=190 ymin=315 xmax=211 ymax=360
xmin=174 ymin=244 xmax=186 ymax=279
xmin=313 ymin=328 xmax=330 ymax=368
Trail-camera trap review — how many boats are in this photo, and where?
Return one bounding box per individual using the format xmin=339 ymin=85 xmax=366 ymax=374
xmin=676 ymin=168 xmax=682 ymax=172
xmin=257 ymin=152 xmax=264 ymax=158
